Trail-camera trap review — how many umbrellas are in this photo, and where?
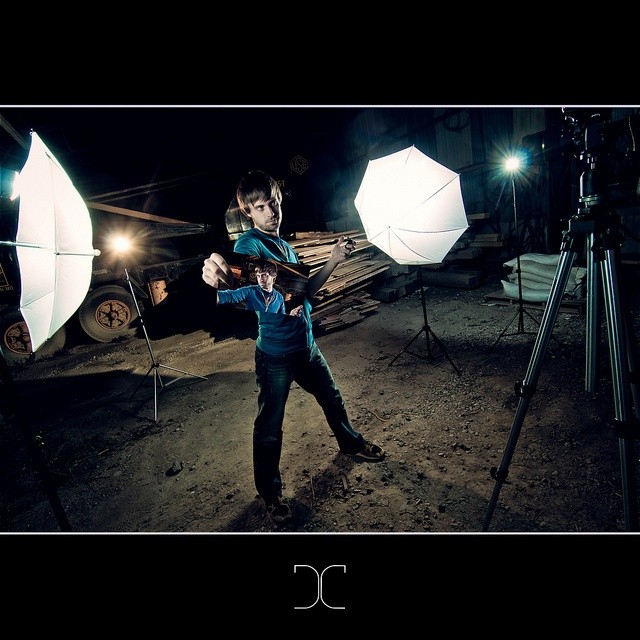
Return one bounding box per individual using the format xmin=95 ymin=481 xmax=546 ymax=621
xmin=339 ymin=143 xmax=473 ymax=266
xmin=0 ymin=128 xmax=102 ymax=357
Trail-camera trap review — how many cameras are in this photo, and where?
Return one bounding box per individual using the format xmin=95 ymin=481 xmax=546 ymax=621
xmin=521 ymin=106 xmax=639 ymax=196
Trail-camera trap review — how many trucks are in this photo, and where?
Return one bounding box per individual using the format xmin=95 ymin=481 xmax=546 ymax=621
xmin=1 ymin=113 xmax=223 ymax=364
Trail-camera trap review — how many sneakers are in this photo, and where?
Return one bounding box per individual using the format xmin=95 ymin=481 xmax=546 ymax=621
xmin=345 ymin=441 xmax=385 ymax=460
xmin=261 ymin=494 xmax=293 ymax=522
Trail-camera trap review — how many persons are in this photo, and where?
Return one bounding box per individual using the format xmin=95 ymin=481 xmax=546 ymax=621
xmin=200 ymin=172 xmax=386 ymax=525
xmin=215 ymin=260 xmax=303 ymax=316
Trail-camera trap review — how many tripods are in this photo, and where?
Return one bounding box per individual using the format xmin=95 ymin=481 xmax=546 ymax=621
xmin=483 ymin=199 xmax=638 ymax=533
